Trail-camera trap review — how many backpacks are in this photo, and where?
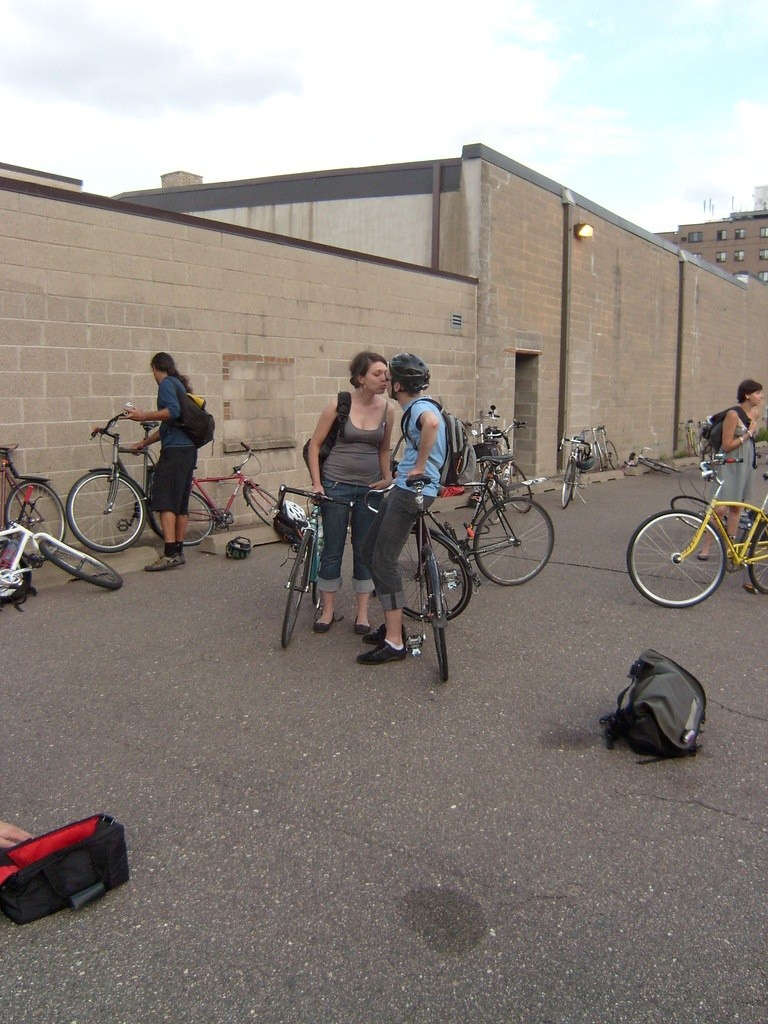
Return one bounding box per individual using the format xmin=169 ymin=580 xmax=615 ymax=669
xmin=0 ymin=548 xmax=38 ymax=612
xmin=302 ymin=391 xmax=351 ymax=484
xmin=400 ymin=399 xmax=476 ymax=487
xmin=158 ymin=376 xmax=215 ymax=449
xmin=709 ymin=406 xmax=758 ymax=469
xmin=473 ymin=441 xmax=508 ymax=474
xmin=273 ymin=511 xmax=310 ymax=545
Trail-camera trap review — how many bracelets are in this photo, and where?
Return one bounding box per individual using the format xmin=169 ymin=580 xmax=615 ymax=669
xmin=747 ymin=431 xmax=753 ymax=437
xmin=739 ymin=437 xmax=744 ymax=444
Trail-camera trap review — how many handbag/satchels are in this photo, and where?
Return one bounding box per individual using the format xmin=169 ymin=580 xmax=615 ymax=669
xmin=599 ymin=650 xmax=707 ymax=765
xmin=0 ymin=813 xmax=130 ymax=925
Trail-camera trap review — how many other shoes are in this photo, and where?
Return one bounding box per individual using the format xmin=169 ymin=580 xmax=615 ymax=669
xmin=155 ymin=553 xmax=185 ymax=567
xmin=145 ymin=553 xmax=182 ymax=571
xmin=697 ymin=550 xmax=710 ymax=561
xmin=357 ymin=641 xmax=406 ymax=665
xmin=354 ymin=616 xmax=371 ymax=635
xmin=362 ymin=624 xmax=406 ymax=645
xmin=313 ymin=609 xmax=334 ymax=633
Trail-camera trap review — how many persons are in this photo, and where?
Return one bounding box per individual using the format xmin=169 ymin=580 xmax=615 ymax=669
xmin=307 ymin=352 xmax=395 ymax=636
xmin=697 ymin=379 xmax=764 ymax=573
xmin=355 ymin=352 xmax=446 ymax=666
xmin=118 ymin=353 xmax=198 ymax=571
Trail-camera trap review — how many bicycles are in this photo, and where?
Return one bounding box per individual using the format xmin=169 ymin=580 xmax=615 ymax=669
xmin=145 ymin=442 xmax=279 ymax=547
xmin=0 ymin=485 xmax=124 ymax=605
xmin=272 ymin=484 xmax=360 ymax=650
xmin=624 ymin=447 xmax=682 ymax=475
xmin=392 ymin=456 xmax=555 ymax=586
xmin=0 ymin=443 xmax=67 ymax=562
xmin=678 ymin=418 xmax=712 ymax=462
xmin=625 ymin=452 xmax=768 ymax=608
xmin=65 ymin=412 xmax=215 ymax=554
xmin=581 ymin=425 xmax=620 ymax=471
xmin=363 ymin=474 xmax=473 ymax=682
xmin=462 ymin=404 xmax=532 ymax=526
xmin=559 ymin=435 xmax=591 ymax=510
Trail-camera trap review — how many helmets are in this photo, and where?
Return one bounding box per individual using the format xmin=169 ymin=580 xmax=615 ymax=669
xmin=468 ymin=492 xmax=487 ymax=507
xmin=576 ymin=456 xmax=596 ymax=470
xmin=389 ymin=353 xmax=431 ymax=394
xmin=225 ymin=537 xmax=252 ymax=559
xmin=282 ymin=501 xmax=306 ymax=522
